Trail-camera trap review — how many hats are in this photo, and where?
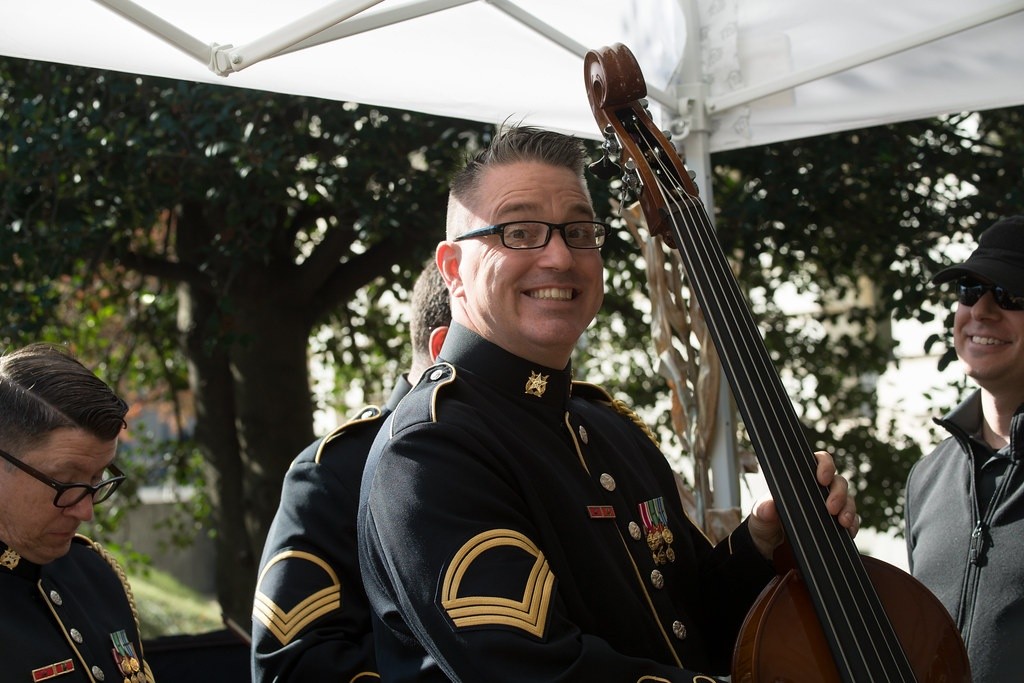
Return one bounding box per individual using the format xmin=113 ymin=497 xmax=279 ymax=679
xmin=932 ymin=217 xmax=1024 ymax=296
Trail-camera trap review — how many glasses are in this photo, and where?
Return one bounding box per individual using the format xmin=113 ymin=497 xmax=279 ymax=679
xmin=955 ymin=277 xmax=1024 ymax=311
xmin=453 ymin=220 xmax=611 ymax=250
xmin=0 ymin=449 xmax=126 ymax=508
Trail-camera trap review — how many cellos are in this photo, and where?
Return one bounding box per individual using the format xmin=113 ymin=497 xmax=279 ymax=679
xmin=581 ymin=40 xmax=974 ymax=683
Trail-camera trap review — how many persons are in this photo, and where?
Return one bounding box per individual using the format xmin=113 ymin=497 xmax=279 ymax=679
xmin=0 ymin=344 xmax=166 ymax=683
xmin=249 ymin=131 xmax=860 ymax=683
xmin=904 ymin=215 xmax=1023 ymax=682
